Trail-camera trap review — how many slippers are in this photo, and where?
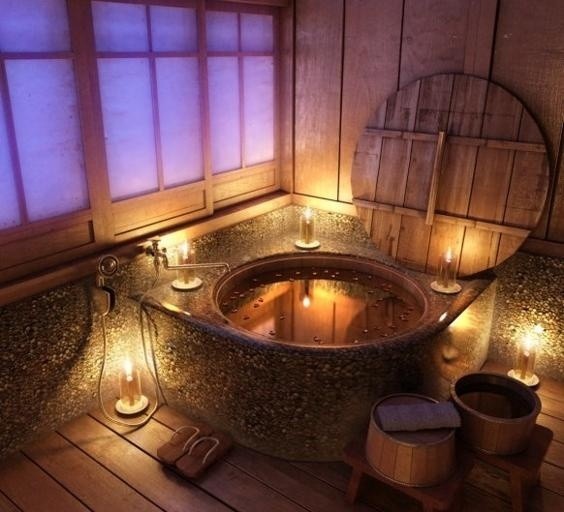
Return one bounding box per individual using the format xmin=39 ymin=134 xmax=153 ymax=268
xmin=176 ymin=432 xmax=232 ymax=476
xmin=157 ymin=422 xmax=215 ymax=464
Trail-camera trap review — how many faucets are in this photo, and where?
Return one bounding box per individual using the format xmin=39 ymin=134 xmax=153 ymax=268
xmin=147 ymin=234 xmax=230 ymax=275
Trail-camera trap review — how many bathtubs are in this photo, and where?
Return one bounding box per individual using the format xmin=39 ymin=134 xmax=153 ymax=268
xmin=211 ymin=253 xmax=428 ymax=348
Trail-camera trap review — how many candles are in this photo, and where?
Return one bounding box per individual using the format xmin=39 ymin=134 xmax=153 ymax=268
xmin=170 ymin=238 xmax=203 ymax=290
xmin=296 ymin=210 xmax=321 ymax=249
xmin=429 ymin=247 xmax=462 ymax=294
xmin=507 ymin=324 xmax=544 ymax=387
xmin=113 ymin=357 xmax=149 ymax=416
